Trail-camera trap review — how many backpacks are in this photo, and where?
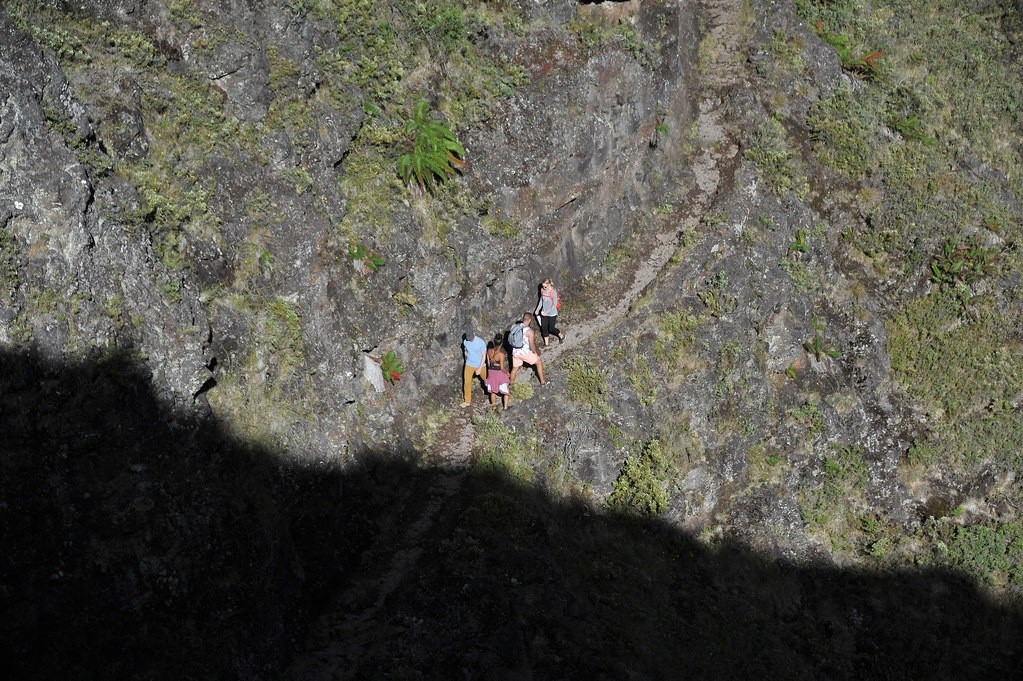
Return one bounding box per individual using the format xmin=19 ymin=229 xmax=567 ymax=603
xmin=507 ymin=323 xmax=528 ymax=348
xmin=551 ymin=289 xmax=561 ymax=310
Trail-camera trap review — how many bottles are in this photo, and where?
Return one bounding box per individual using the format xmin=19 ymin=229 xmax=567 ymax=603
xmin=536 ymin=314 xmax=542 ymax=326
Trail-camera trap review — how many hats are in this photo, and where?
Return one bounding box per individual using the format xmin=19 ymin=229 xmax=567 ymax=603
xmin=465 ymin=329 xmax=474 ymax=340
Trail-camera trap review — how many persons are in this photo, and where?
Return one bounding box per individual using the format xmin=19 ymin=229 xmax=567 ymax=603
xmin=535 ymin=278 xmax=565 ymax=349
xmin=485 ymin=333 xmax=513 ymax=410
xmin=460 ymin=329 xmax=487 ymax=407
xmin=509 ymin=311 xmax=547 ymax=385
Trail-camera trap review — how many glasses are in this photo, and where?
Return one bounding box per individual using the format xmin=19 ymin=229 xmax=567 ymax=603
xmin=543 ymin=284 xmax=550 ymax=288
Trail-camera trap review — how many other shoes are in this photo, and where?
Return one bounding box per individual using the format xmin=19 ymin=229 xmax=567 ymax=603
xmin=539 ymin=343 xmax=550 ymax=350
xmin=541 ymin=380 xmax=550 ymax=387
xmin=460 ymin=402 xmax=471 ymax=408
xmin=559 ymin=335 xmax=566 ymax=345
